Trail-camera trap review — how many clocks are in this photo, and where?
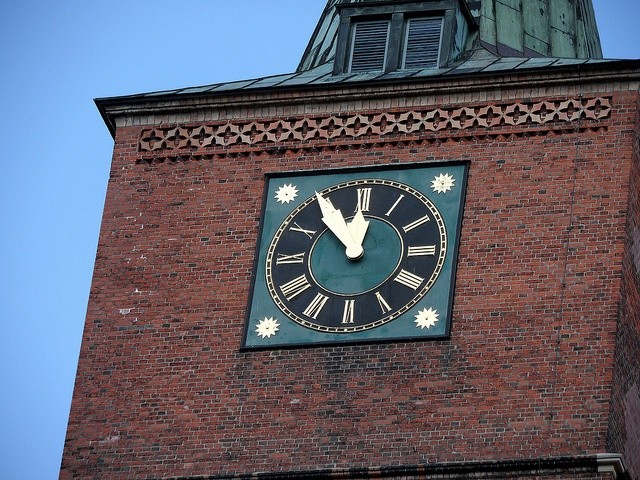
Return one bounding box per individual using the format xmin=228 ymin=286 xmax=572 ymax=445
xmin=239 ymin=156 xmax=470 ymax=350
